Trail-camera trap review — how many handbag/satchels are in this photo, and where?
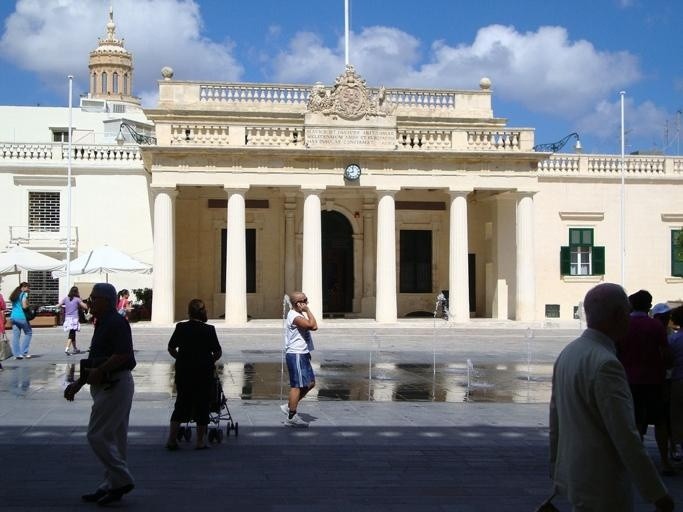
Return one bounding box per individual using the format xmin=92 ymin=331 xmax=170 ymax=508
xmin=1 ymin=339 xmax=13 ymax=361
xmin=118 ymin=308 xmax=127 ymax=316
xmin=23 ymin=306 xmax=36 ymax=320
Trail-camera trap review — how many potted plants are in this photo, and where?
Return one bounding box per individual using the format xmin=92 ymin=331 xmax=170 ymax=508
xmin=131 ymin=288 xmax=152 ymax=318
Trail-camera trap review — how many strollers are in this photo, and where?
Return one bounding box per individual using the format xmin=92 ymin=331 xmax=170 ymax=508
xmin=177 ymin=354 xmax=238 ymax=444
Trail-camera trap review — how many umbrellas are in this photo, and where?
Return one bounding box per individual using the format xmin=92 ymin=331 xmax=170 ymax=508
xmin=0 ymin=245 xmax=64 ymax=283
xmin=50 ymin=243 xmax=153 ymax=284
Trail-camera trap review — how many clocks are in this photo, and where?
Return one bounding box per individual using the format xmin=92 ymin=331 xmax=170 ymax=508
xmin=345 ymin=163 xmax=361 ymax=181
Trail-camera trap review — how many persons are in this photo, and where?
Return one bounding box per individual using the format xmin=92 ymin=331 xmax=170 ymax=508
xmin=62 ymin=282 xmax=137 ymax=508
xmin=114 ymin=289 xmax=132 ymax=324
xmin=165 ymin=298 xmax=223 ymax=452
xmin=547 ymin=282 xmax=682 ymax=511
xmin=279 ymin=291 xmax=318 ymax=429
xmin=0 ymin=292 xmax=7 ymax=369
xmin=55 ymin=286 xmax=88 ymax=356
xmin=7 ymin=282 xmax=32 ymax=360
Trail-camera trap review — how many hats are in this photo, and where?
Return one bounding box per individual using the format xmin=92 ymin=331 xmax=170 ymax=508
xmin=653 ymin=303 xmax=671 ymax=315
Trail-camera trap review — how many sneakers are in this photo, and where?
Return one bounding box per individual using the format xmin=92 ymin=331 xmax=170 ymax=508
xmin=165 ymin=443 xmax=179 ymax=449
xmin=285 ymin=413 xmax=308 ymax=428
xmin=281 ymin=403 xmax=290 ymax=414
xmin=194 ymin=444 xmax=209 ymax=449
xmin=64 ymin=348 xmax=71 ymax=355
xmin=81 ymin=485 xmax=134 ymax=505
xmin=73 ymin=347 xmax=80 ymax=353
